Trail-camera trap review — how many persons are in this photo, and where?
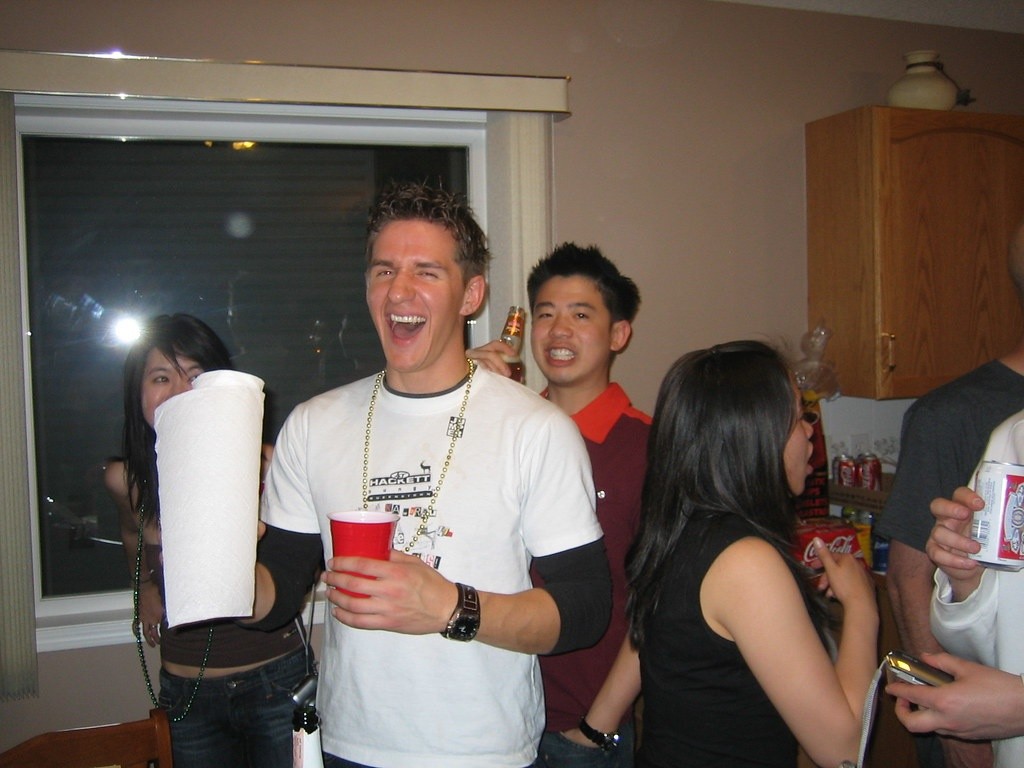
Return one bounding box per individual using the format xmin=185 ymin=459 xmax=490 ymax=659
xmin=232 ymin=174 xmax=613 ymax=768
xmin=873 ymin=327 xmax=1024 ymax=768
xmin=100 ymin=312 xmax=318 ymax=768
xmin=885 ymin=408 xmax=1024 ymax=768
xmin=622 ymin=339 xmax=880 ymax=768
xmin=464 ymin=239 xmax=654 ymax=768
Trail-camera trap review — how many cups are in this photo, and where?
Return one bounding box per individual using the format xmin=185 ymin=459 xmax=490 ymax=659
xmin=326 ymin=509 xmax=400 ymax=599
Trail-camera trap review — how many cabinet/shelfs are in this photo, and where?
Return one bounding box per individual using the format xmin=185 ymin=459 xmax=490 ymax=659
xmin=806 ymin=105 xmax=1024 ymax=400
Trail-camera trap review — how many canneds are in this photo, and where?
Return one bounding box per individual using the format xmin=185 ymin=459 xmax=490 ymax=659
xmin=968 ymin=460 xmax=1024 ymax=572
xmin=833 ymin=451 xmax=881 ymax=491
xmin=840 ymin=504 xmax=887 ymax=575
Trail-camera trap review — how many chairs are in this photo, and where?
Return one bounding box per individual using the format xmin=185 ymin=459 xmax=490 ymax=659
xmin=0 ymin=706 xmax=173 ymax=768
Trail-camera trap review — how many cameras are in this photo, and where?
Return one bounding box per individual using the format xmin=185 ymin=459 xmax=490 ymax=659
xmin=885 ymin=650 xmax=955 ymax=689
xmin=291 ymin=676 xmax=320 ymax=733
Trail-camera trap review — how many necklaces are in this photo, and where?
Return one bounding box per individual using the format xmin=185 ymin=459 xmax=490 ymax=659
xmin=134 ymin=474 xmax=213 ymax=721
xmin=362 ymin=357 xmax=474 ymax=552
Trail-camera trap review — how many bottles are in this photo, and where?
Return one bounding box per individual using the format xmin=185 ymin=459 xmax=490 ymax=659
xmin=889 ymin=51 xmax=958 ymax=110
xmin=841 ymin=505 xmax=889 ymax=572
xmin=496 ymin=305 xmax=526 ymax=384
xmin=291 ymin=705 xmax=325 ymax=768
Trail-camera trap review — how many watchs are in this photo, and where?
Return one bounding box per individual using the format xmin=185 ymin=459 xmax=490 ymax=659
xmin=441 ymin=583 xmax=480 ymax=642
xmin=580 ymin=716 xmax=619 ymax=752
